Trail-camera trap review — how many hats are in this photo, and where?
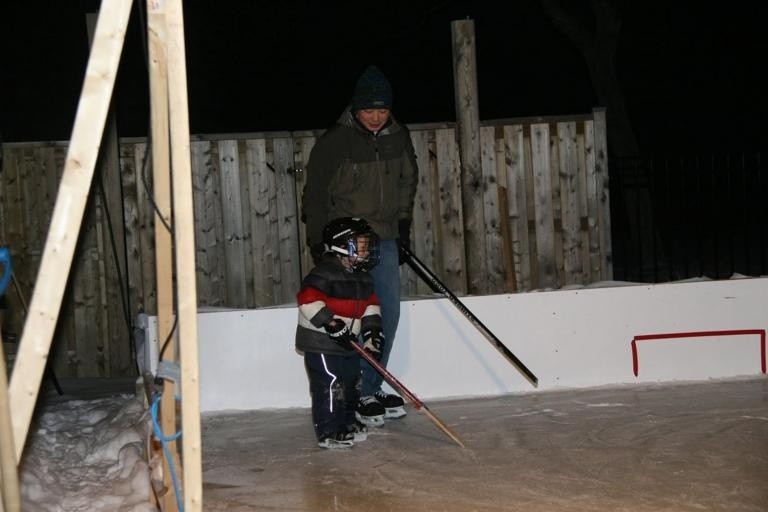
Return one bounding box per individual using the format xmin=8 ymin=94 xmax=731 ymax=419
xmin=349 ymin=64 xmax=395 ymax=110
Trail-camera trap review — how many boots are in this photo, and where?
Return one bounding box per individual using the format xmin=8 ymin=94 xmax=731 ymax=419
xmin=318 ymin=389 xmax=404 ymax=442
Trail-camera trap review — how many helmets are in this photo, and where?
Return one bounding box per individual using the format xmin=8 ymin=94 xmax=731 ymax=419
xmin=321 ymin=216 xmax=381 ymax=254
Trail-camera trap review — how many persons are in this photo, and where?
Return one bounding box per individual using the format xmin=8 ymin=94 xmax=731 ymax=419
xmin=300 ymin=63 xmax=420 ymax=421
xmin=291 ymin=217 xmax=386 ymax=450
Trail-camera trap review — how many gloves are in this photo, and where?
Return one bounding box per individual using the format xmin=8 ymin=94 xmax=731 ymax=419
xmin=363 ymin=331 xmax=385 ymax=361
xmin=323 ymin=317 xmax=358 ymax=347
xmin=399 ymin=232 xmax=411 ymax=266
xmin=310 ymin=242 xmax=326 ymax=267
